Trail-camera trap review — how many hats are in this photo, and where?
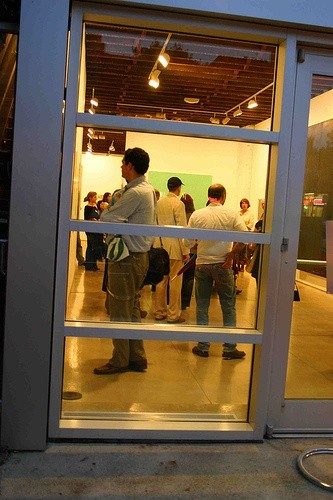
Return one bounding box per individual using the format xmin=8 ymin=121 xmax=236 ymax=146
xmin=168 ymin=177 xmax=185 ymax=187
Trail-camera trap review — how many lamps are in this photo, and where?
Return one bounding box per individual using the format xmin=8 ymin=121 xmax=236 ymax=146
xmin=90 ymin=97 xmax=99 ymax=107
xmin=109 ymin=140 xmax=115 ymax=152
xmin=88 ymin=108 xmax=96 ymax=115
xmin=149 ymin=69 xmax=161 ymax=89
xmin=247 ymin=96 xmax=258 ymax=109
xmin=234 ymin=106 xmax=243 ymax=117
xmin=210 ymin=113 xmax=220 ymax=124
xmin=222 ymin=112 xmax=231 ymax=125
xmin=87 ymin=128 xmax=105 ymax=152
xmin=158 ymin=49 xmax=171 ymax=68
xmin=156 ymin=108 xmax=166 ymax=118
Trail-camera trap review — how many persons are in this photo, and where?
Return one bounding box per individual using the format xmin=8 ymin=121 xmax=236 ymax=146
xmin=140 ymin=188 xmax=160 ymax=319
xmin=206 ymin=200 xmax=242 ymax=295
xmin=102 ymin=189 xmax=122 ymax=314
xmin=94 ymin=147 xmax=157 ymax=375
xmin=236 ymin=198 xmax=255 ymax=272
xmin=167 ymin=193 xmax=198 ymax=310
xmin=249 ymin=197 xmax=265 ymax=278
xmin=97 ymin=192 xmax=112 ymax=262
xmin=183 ymin=183 xmax=249 ymax=358
xmin=83 ymin=191 xmax=100 ymax=271
xmin=150 ymin=177 xmax=190 ymax=323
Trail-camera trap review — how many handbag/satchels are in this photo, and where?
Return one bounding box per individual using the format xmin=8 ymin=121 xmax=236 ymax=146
xmin=294 ymin=290 xmax=299 ymax=301
xmin=143 ymin=247 xmax=170 ymax=286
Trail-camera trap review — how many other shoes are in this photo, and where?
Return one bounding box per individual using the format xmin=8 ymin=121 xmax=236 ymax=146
xmin=192 ymin=346 xmax=209 ymax=357
xmin=235 ymin=289 xmax=241 ymax=293
xmin=85 ymin=266 xmax=101 ymax=271
xmin=141 ymin=311 xmax=147 ymax=318
xmin=94 ymin=362 xmax=129 ymax=374
xmin=169 ymin=316 xmax=185 ymax=323
xmin=222 ymin=348 xmax=246 ymax=358
xmin=129 ymin=362 xmax=148 ymax=369
